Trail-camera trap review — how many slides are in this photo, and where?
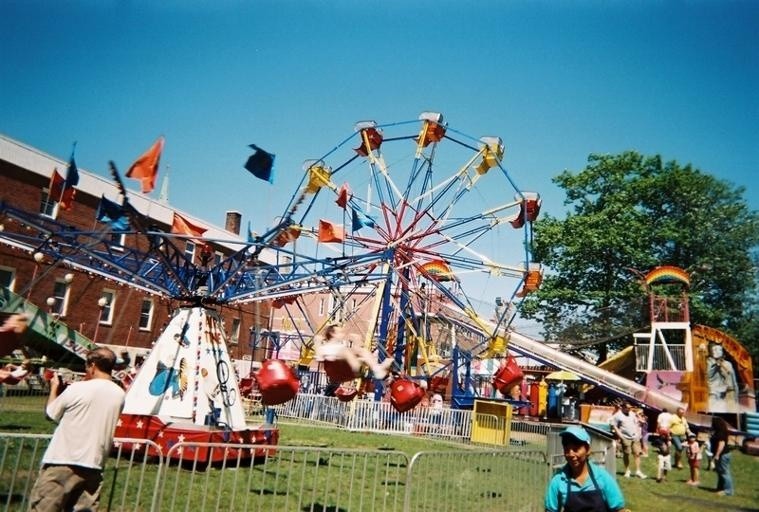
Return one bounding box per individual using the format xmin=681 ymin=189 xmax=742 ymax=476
xmin=436 ymin=299 xmax=742 ymax=435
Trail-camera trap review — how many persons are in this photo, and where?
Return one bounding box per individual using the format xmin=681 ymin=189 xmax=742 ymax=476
xmin=609 ymin=400 xmax=713 ymax=486
xmin=289 ymin=380 xmax=445 ymax=437
xmin=710 ymin=416 xmax=734 ymax=496
xmin=419 ymin=281 xmax=427 ymax=311
xmin=27 ymin=346 xmax=127 ymax=511
xmin=113 ymin=351 xmax=130 ymax=371
xmin=0 ymin=311 xmax=30 ymax=334
xmin=0 ymin=358 xmax=31 ymax=384
xmin=542 ymin=424 xmax=632 ymax=512
xmin=313 ymin=323 xmax=393 ymax=382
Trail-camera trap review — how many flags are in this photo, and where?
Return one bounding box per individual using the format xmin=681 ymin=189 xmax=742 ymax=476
xmin=169 ymin=211 xmax=208 ymax=247
xmin=243 ymin=143 xmax=277 ymax=182
xmin=46 ymin=169 xmax=78 ymax=212
xmin=335 ymin=186 xmax=364 ymax=235
xmin=95 ymin=195 xmax=133 ymax=234
xmin=124 ymin=135 xmax=165 ymax=193
xmin=159 ymin=170 xmax=170 ymax=205
xmin=62 ymin=153 xmax=79 ymax=192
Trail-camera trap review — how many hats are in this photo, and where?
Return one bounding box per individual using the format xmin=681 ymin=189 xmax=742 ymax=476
xmin=559 ymin=425 xmax=591 ymax=445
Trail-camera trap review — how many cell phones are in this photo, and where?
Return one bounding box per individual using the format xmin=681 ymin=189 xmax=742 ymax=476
xmin=58 ymin=373 xmax=62 ymax=382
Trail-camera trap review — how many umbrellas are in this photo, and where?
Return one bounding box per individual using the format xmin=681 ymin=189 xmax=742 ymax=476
xmin=544 ymin=369 xmax=582 ymax=384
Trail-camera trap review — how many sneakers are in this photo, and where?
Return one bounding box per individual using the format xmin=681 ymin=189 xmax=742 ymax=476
xmin=636 ymin=470 xmax=648 ymax=479
xmin=717 ymin=490 xmax=726 ymax=497
xmin=624 ymin=469 xmax=631 ymax=478
xmin=686 ymin=479 xmax=700 ymax=485
